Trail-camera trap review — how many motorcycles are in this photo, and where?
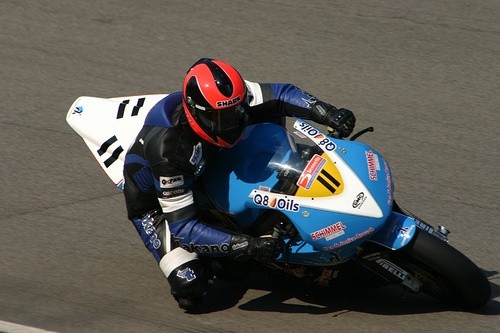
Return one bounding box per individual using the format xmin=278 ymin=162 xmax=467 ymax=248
xmin=65 ymin=96 xmax=492 ymax=312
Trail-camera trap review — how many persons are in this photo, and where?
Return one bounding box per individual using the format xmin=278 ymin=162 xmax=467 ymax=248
xmin=123 ymin=56 xmax=355 ymax=313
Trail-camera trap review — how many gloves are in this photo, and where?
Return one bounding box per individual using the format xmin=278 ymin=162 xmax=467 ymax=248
xmin=310 ymin=101 xmax=357 ymax=139
xmin=228 ymin=234 xmax=286 ymax=263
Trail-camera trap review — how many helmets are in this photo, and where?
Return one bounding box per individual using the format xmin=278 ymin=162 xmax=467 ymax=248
xmin=181 ymin=57 xmax=254 ymax=148
